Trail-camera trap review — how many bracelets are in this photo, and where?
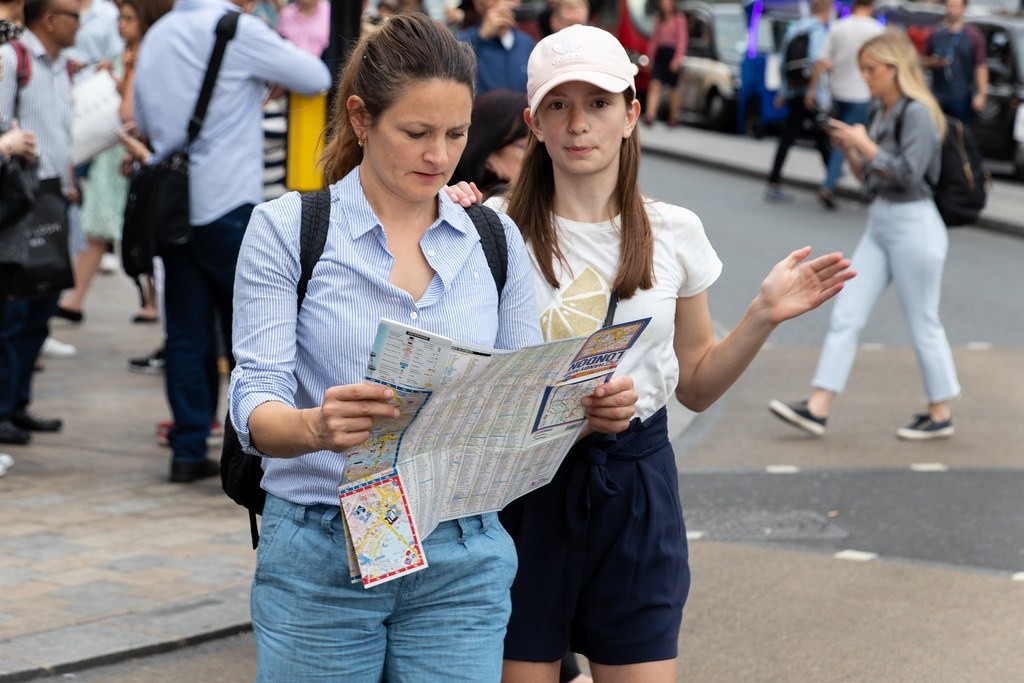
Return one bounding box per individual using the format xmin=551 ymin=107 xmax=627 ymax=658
xmin=1 ymin=135 xmax=14 ymax=158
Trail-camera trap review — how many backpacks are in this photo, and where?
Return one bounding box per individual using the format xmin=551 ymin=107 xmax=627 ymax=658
xmin=868 ymin=98 xmax=986 ymax=227
xmin=783 ymin=33 xmax=812 ymax=89
xmin=220 ymin=190 xmax=508 ymax=514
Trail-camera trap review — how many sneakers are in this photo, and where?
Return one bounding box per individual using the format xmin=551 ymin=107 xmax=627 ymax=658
xmin=769 ymin=399 xmax=828 ymax=435
xmin=897 ymin=414 xmax=957 ymax=440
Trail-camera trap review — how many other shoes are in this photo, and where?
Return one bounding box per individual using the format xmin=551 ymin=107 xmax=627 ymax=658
xmin=14 ymin=413 xmax=61 ymax=438
xmin=170 ymin=459 xmax=219 ymax=482
xmin=1 ymin=421 xmax=29 ymax=444
xmin=42 ymin=338 xmax=77 ymax=359
xmin=99 ymin=253 xmax=117 ymax=272
xmin=0 ymin=453 xmax=15 ymax=476
xmin=54 ymin=305 xmax=83 ymax=321
xmin=764 ymin=189 xmax=795 ymax=202
xmin=134 ymin=315 xmax=162 ymax=322
xmin=156 ymin=422 xmax=223 ymax=446
xmin=129 ymin=350 xmax=167 ymax=375
xmin=816 ymin=188 xmax=842 ymax=210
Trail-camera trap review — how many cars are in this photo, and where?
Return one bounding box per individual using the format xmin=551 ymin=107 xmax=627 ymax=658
xmin=870 ymin=0 xmax=1024 ymax=174
xmin=650 ymin=0 xmax=781 ymax=136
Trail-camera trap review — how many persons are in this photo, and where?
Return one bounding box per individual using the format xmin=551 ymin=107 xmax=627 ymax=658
xmin=0 ymin=0 xmax=591 ymax=477
xmin=767 ymin=0 xmax=988 ymax=212
xmin=133 ymin=1 xmax=333 ymax=484
xmin=642 ymin=0 xmax=689 ymax=127
xmin=228 ymin=12 xmax=520 ymax=683
xmin=442 ymin=22 xmax=857 ymax=683
xmin=445 ymin=88 xmax=596 ymax=683
xmin=767 ymin=29 xmax=962 ymax=442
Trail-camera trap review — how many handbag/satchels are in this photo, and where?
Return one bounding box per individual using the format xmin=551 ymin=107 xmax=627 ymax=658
xmin=118 ymin=153 xmax=191 ymax=276
xmin=0 ymin=141 xmax=77 ymax=292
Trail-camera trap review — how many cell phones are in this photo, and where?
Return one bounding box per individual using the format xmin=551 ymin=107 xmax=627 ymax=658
xmin=512 ymin=7 xmax=533 ymax=20
xmin=816 ymin=115 xmax=834 ymax=129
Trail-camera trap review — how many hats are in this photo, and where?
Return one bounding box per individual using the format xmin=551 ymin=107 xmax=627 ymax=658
xmin=526 ymin=24 xmax=639 ymax=119
